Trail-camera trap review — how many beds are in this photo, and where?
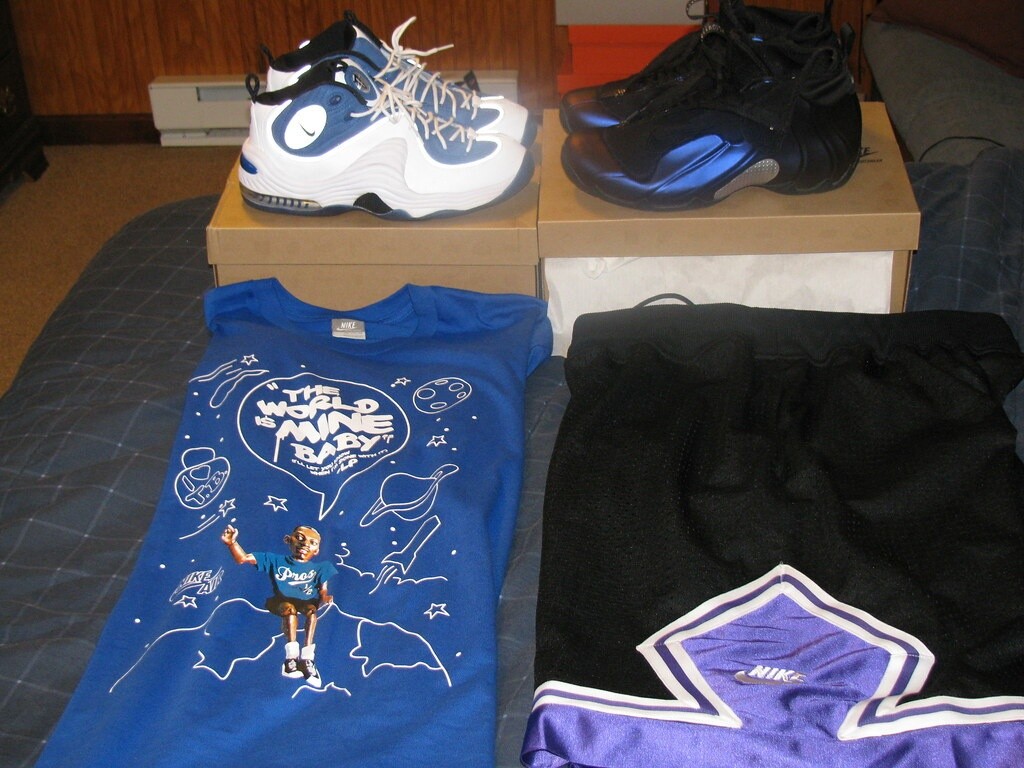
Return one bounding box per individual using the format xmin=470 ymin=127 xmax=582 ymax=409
xmin=0 ymin=150 xmax=1024 ymax=768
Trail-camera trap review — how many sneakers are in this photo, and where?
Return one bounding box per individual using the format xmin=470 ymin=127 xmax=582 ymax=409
xmin=238 ymin=59 xmax=533 ymax=222
xmin=260 ymin=11 xmax=537 ymax=150
xmin=561 ymin=0 xmax=835 ymax=134
xmin=560 ymin=23 xmax=861 ymax=211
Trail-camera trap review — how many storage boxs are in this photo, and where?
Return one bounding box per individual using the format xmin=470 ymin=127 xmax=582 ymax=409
xmin=537 ymin=102 xmax=922 ymax=360
xmin=555 ymin=0 xmax=707 ymax=98
xmin=204 ymin=116 xmax=540 ymax=311
